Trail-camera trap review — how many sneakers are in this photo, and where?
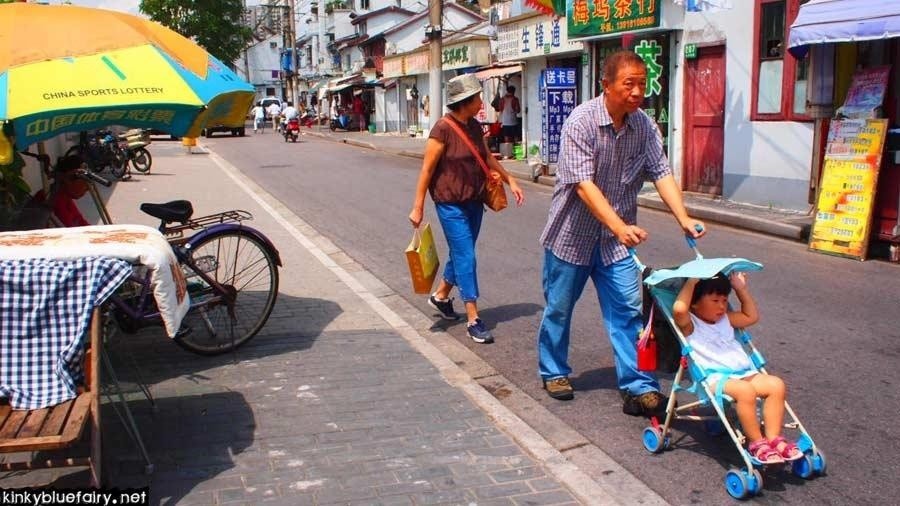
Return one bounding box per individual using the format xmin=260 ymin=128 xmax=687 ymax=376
xmin=543 ymin=377 xmax=574 ymax=400
xmin=427 ymin=291 xmax=460 ymax=320
xmin=623 ymin=389 xmax=678 ymax=417
xmin=465 ymin=318 xmax=493 ymax=343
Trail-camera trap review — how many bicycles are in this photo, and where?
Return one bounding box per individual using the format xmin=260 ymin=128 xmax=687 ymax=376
xmin=18 ymin=150 xmax=283 ymax=358
xmin=251 ymin=111 xmax=280 ymax=134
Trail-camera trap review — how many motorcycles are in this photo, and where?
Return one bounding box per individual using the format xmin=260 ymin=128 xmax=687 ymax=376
xmin=1 ymin=123 xmax=32 ymax=227
xmin=330 ymin=113 xmax=352 ymax=131
xmin=66 ymin=127 xmax=152 ymax=178
xmin=278 ymin=110 xmax=300 ymax=142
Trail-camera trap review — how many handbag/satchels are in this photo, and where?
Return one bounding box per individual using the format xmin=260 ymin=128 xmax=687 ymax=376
xmin=404 ymin=222 xmax=439 ymax=294
xmin=636 ymin=300 xmax=661 ymax=371
xmin=491 ymin=93 xmax=500 ymax=112
xmin=484 ymin=167 xmax=508 ymax=212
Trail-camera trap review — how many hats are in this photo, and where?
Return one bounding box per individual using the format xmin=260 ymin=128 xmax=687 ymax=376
xmin=446 ymin=72 xmax=484 ymax=107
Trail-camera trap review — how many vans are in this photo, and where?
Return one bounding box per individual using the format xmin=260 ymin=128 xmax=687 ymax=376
xmin=258 ymin=96 xmax=282 ymax=119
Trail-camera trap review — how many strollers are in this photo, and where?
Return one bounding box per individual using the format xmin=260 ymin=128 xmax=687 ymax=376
xmin=625 ymin=224 xmax=827 ymax=498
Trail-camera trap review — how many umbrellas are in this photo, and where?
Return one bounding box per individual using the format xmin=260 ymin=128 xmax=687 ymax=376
xmin=0 ymin=2 xmax=259 ymax=165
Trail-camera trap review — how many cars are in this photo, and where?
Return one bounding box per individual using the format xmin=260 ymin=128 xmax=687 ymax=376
xmin=202 ymin=125 xmax=246 ymax=137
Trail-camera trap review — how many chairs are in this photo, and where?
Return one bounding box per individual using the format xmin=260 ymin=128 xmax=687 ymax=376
xmin=116 ymin=255 xmax=224 ymax=339
xmin=0 ymin=258 xmax=106 ymax=493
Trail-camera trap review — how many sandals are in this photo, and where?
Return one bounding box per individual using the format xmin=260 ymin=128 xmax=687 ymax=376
xmin=748 ymin=436 xmax=785 ymax=464
xmin=769 ymin=435 xmax=804 ymax=461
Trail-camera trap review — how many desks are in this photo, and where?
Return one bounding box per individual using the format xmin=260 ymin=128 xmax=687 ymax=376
xmin=86 ymin=308 xmax=159 ymax=478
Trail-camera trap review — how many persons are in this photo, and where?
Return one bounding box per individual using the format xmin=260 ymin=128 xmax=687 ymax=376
xmin=408 ymin=72 xmax=526 ymax=345
xmin=28 ymin=153 xmax=98 ymax=229
xmin=671 ymin=269 xmax=806 ymax=466
xmin=496 ymin=84 xmax=521 ymax=143
xmin=534 ymin=49 xmax=708 ymax=416
xmin=248 ymin=94 xmax=369 ymax=142
